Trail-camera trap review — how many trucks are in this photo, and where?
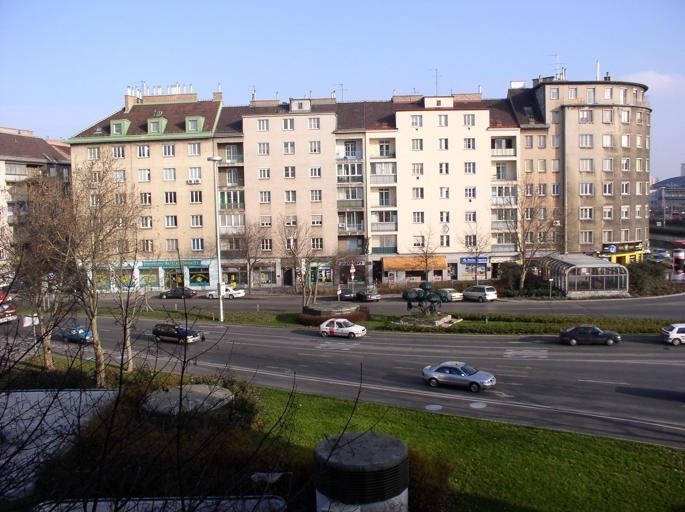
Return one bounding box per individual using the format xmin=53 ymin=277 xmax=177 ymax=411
xmin=0 ymin=293 xmax=17 ymax=324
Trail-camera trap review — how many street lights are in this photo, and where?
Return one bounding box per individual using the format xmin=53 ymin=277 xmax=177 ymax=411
xmin=661 ymin=187 xmax=666 ymax=227
xmin=206 ymin=156 xmax=223 ymax=322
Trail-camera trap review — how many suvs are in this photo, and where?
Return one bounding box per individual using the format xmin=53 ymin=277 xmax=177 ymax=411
xmin=151 ymin=322 xmax=200 ymax=344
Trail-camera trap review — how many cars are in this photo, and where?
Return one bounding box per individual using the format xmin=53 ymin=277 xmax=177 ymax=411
xmin=62 ymin=325 xmax=94 ymax=344
xmin=205 ymin=285 xmax=245 ymax=300
xmin=647 ymin=248 xmax=671 ymax=265
xmin=160 ymin=286 xmax=198 ymax=298
xmin=356 ymin=288 xmax=382 ymax=302
xmin=434 ymin=288 xmax=463 ymax=303
xmin=661 ymin=323 xmax=685 ymax=346
xmin=671 ymin=211 xmax=685 ymax=222
xmin=559 ymin=324 xmax=622 ymax=346
xmin=422 ymin=361 xmax=497 ymax=392
xmin=337 ymin=289 xmax=355 ymax=302
xmin=319 ymin=318 xmax=367 ymax=339
xmin=406 ymin=287 xmax=427 ymax=302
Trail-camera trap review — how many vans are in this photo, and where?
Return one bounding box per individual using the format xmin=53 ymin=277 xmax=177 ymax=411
xmin=461 ymin=285 xmax=498 ymax=303
